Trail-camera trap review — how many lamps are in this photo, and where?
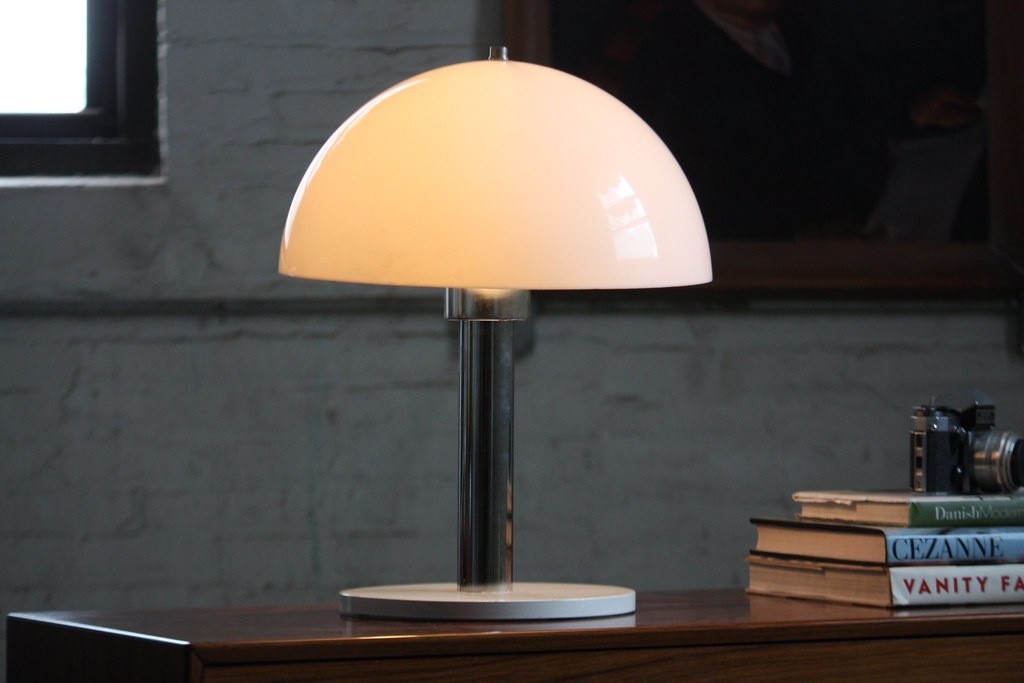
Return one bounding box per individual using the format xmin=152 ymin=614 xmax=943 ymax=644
xmin=276 ymin=45 xmax=715 ymax=625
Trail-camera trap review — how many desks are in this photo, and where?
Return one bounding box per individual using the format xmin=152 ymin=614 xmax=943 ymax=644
xmin=7 ymin=588 xmax=1024 ymax=683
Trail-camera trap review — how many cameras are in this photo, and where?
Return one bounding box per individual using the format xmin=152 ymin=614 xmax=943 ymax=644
xmin=910 ymin=402 xmax=1024 ymax=497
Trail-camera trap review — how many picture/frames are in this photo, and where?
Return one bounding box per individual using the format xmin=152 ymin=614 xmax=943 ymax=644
xmin=492 ymin=0 xmax=1024 ymax=306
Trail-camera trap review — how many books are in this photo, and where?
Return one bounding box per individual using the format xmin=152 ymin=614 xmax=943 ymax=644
xmin=742 ymin=490 xmax=1024 ymax=608
